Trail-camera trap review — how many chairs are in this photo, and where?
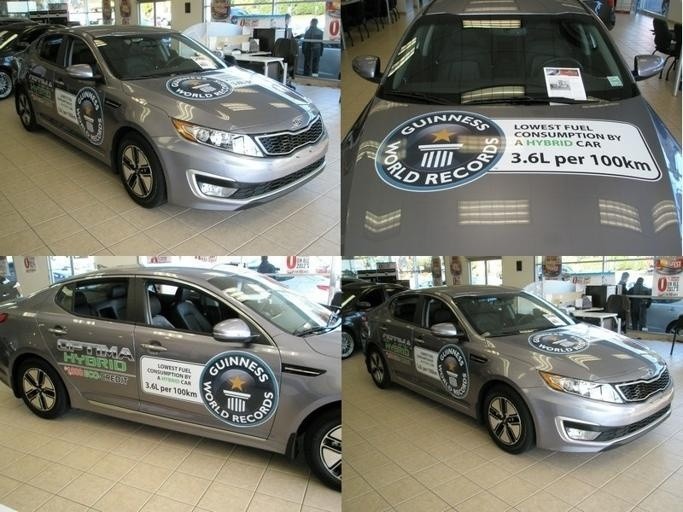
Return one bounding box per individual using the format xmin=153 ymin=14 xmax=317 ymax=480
xmin=428 ymin=309 xmax=451 ymax=330
xmin=514 ymin=20 xmax=570 ymax=78
xmin=651 ymin=18 xmax=681 ymax=90
xmin=398 ymin=303 xmax=416 ymax=322
xmin=148 ymin=286 xmax=212 ymax=332
xmin=669 ymin=314 xmax=682 ymax=355
xmin=70 ymin=286 xmax=126 ymax=320
xmin=271 ymin=38 xmax=297 ymax=90
xmin=123 ymin=43 xmax=154 ymax=73
xmin=603 ymin=295 xmax=628 ymax=336
xmin=340 ymin=0 xmax=400 ymax=52
xmin=435 ymin=26 xmax=492 ymax=79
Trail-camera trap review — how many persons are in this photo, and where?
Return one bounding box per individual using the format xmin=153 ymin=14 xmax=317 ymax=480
xmin=628 ymin=277 xmax=652 ymax=331
xmin=285 ymin=14 xmax=304 ymax=39
xmin=257 ymin=256 xmax=275 ymax=273
xmin=615 ymin=272 xmax=629 ymax=295
xmin=302 ymin=19 xmax=323 ymax=77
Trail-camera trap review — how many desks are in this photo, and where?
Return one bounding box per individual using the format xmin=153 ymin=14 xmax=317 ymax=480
xmin=223 ymin=51 xmax=287 ymax=85
xmin=569 ymin=308 xmax=620 ymax=335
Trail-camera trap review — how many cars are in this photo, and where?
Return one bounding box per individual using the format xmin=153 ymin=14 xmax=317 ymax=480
xmin=293 ymin=32 xmax=340 ymax=81
xmin=642 ymin=292 xmax=681 ymax=335
xmin=0 ymin=19 xmax=68 ymax=101
xmin=1 ymin=264 xmax=340 ymax=492
xmin=362 ymin=284 xmax=656 ymax=457
xmin=341 ymin=281 xmax=410 ymax=360
xmin=263 ymin=270 xmax=335 ymax=308
xmin=13 ymin=23 xmax=328 ymax=213
xmin=339 ymin=0 xmax=677 ymax=256
